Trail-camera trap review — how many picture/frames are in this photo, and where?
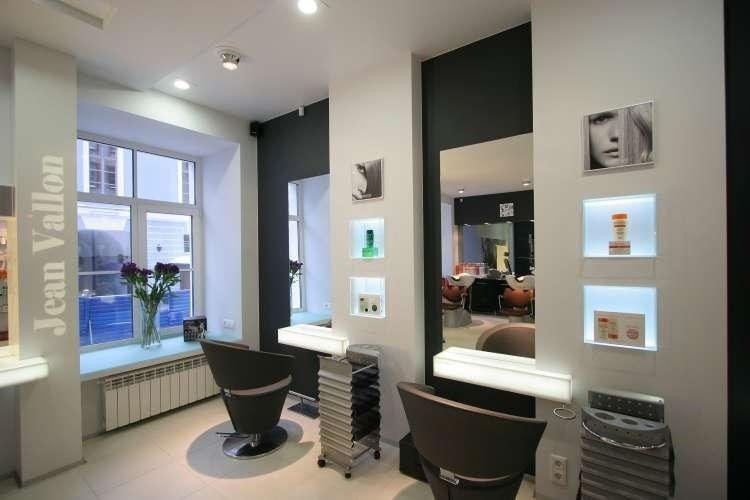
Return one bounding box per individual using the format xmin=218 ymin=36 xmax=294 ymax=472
xmin=351 ymin=158 xmax=385 ymax=205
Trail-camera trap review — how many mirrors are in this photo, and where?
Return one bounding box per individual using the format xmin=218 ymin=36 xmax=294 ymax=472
xmin=458 ymin=220 xmax=517 ymax=279
xmin=439 ymin=132 xmax=534 ymax=354
xmin=286 ymin=174 xmax=335 ymax=329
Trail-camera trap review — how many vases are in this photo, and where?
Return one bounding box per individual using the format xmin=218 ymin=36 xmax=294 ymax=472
xmin=137 ymin=294 xmax=163 ymax=353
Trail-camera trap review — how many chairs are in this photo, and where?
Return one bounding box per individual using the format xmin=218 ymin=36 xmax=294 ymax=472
xmin=196 ymin=337 xmax=298 ymax=461
xmin=396 ymin=380 xmax=550 ymax=500
xmin=440 ymin=272 xmax=535 ymax=330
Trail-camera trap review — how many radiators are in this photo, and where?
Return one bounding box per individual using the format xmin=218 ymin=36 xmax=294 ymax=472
xmin=95 ymin=355 xmax=223 ymax=435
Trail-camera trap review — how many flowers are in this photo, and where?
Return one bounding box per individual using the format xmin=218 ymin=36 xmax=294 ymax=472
xmin=119 ymin=259 xmax=184 ymax=348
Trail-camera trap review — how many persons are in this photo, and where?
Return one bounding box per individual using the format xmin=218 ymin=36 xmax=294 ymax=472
xmin=350 ymin=159 xmax=383 ymax=200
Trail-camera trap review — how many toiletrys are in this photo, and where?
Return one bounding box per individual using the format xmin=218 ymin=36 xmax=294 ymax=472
xmin=611 ymin=214 xmax=628 ymax=240
xmin=363 ymin=230 xmax=377 ymax=258
